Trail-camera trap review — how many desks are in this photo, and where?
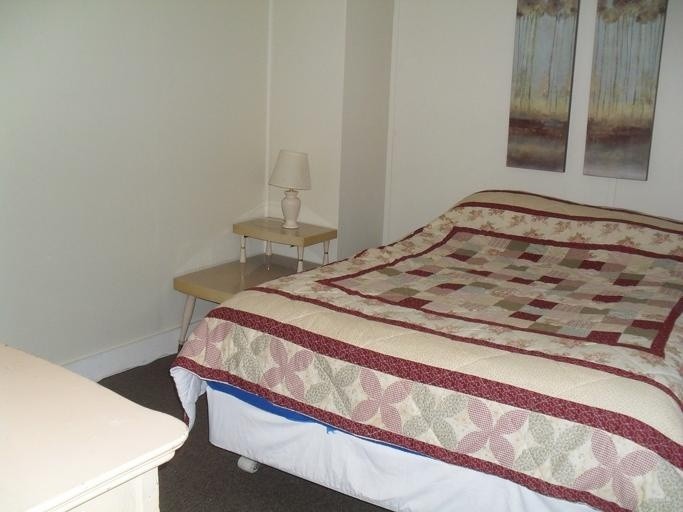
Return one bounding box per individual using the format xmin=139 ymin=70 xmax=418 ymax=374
xmin=0 ymin=342 xmax=190 ymax=512
xmin=172 ymin=252 xmax=324 ymax=352
xmin=233 ymin=215 xmax=337 ymax=275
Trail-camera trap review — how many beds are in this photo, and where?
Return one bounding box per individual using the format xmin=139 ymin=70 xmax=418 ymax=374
xmin=169 ymin=189 xmax=683 ymax=511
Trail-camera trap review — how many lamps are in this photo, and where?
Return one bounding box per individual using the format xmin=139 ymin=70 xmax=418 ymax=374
xmin=267 ymin=150 xmax=313 ymax=230
